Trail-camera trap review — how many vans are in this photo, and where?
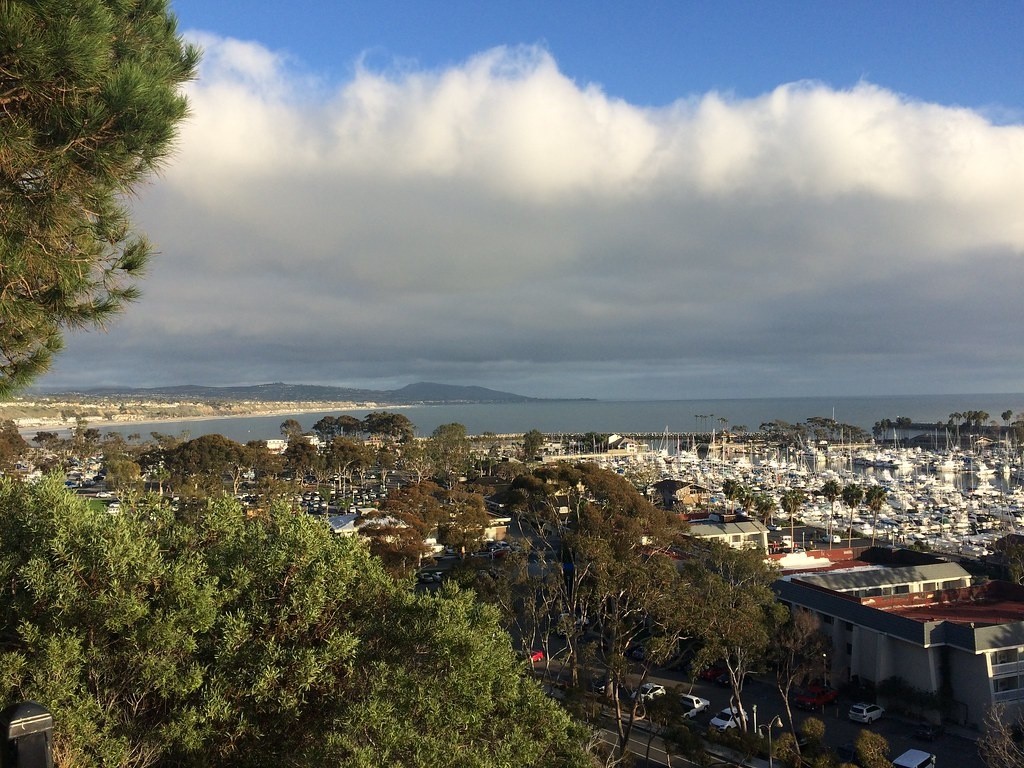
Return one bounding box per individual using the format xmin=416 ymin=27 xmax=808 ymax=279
xmin=892 ymin=749 xmax=935 ymax=768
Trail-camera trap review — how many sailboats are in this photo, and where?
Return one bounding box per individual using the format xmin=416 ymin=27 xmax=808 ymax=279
xmin=606 ymin=424 xmax=1024 ymax=553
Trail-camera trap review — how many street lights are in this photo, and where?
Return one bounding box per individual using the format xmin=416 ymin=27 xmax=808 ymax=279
xmin=757 ymin=715 xmax=784 ymax=768
xmin=822 ymin=653 xmax=827 ymax=686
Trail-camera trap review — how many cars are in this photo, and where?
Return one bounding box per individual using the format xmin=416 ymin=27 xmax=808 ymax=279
xmin=675 ymin=692 xmax=710 ymax=721
xmin=687 ymin=662 xmax=754 ymax=689
xmin=767 ymin=524 xmax=783 ymax=531
xmin=632 ymin=683 xmax=666 ymax=705
xmin=235 ymin=469 xmax=389 ymax=517
xmin=531 ymin=650 xmax=543 ymax=663
xmin=709 ymin=706 xmax=749 ymax=734
xmin=417 ymin=536 xmax=524 ymax=583
xmin=794 ymin=681 xmax=838 ymax=716
xmin=916 ymin=720 xmax=944 ymax=742
xmin=24 ymin=451 xmax=127 ymax=518
xmin=822 ymin=535 xmax=841 ymax=544
xmin=848 ymin=702 xmax=887 ymax=726
xmin=633 ymin=646 xmax=649 ymax=660
xmin=557 ymin=613 xmax=589 ymax=638
